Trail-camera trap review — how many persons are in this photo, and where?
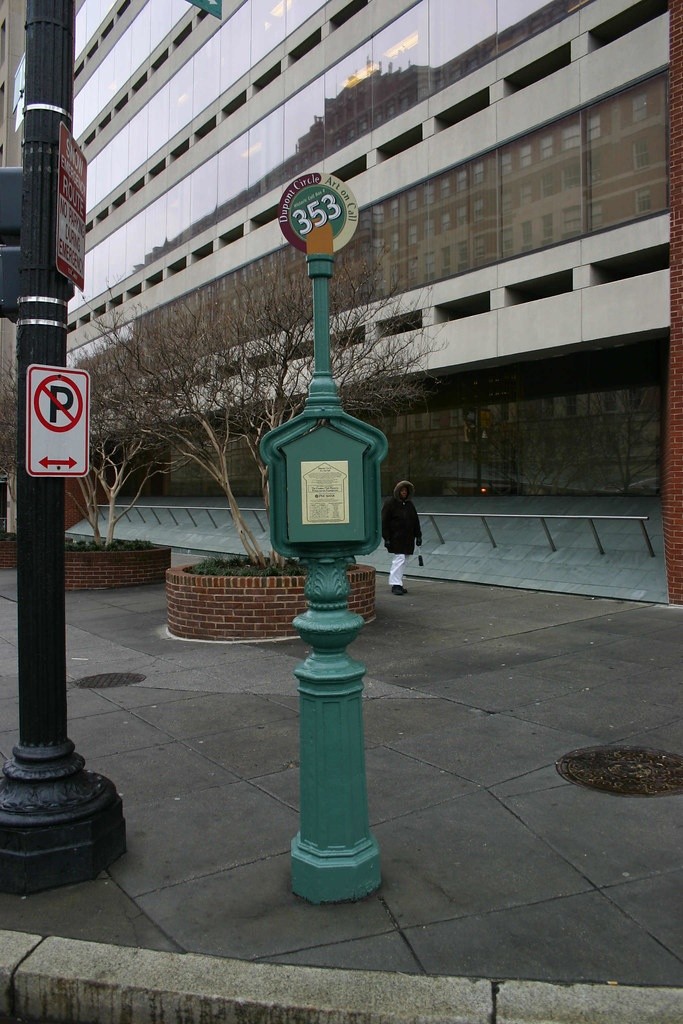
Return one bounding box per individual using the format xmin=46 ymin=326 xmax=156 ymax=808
xmin=381 ymin=479 xmax=422 ymax=595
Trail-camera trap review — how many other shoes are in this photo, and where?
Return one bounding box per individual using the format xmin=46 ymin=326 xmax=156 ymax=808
xmin=401 ymin=588 xmax=407 ymax=593
xmin=392 ymin=585 xmax=403 ymax=595
